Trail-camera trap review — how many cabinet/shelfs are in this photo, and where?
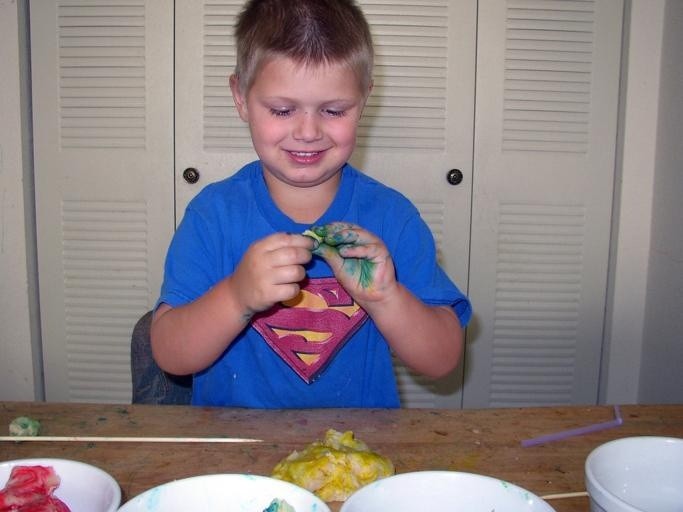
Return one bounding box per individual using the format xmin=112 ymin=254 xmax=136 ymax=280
xmin=27 ymin=0 xmax=622 ymax=406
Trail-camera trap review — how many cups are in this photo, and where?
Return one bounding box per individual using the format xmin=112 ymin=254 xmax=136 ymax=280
xmin=584 ymin=434 xmax=683 ymax=511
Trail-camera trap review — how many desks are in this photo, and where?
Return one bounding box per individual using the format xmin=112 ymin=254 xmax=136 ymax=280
xmin=0 ymin=400 xmax=683 ymax=512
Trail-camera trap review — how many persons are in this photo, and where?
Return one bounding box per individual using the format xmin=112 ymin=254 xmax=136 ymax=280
xmin=148 ymin=0 xmax=476 ymax=408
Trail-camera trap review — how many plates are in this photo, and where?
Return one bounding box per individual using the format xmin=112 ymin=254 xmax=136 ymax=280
xmin=0 ymin=459 xmax=120 ymax=511
xmin=118 ymin=473 xmax=332 ymax=512
xmin=338 ymin=469 xmax=554 ymax=510
